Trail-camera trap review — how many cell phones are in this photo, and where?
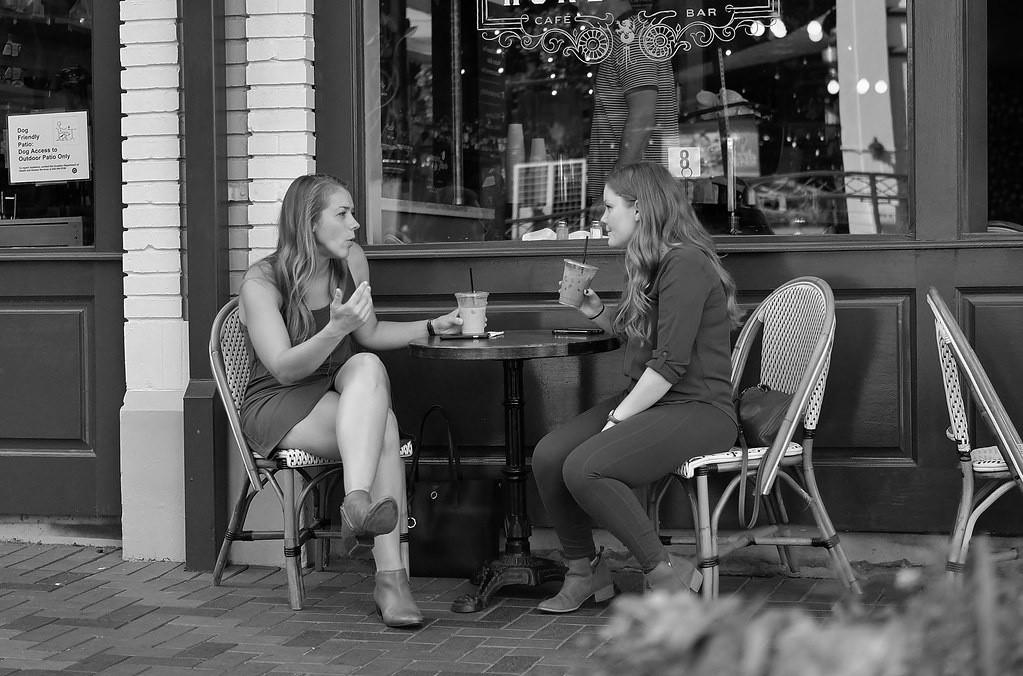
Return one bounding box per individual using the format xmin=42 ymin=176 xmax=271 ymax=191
xmin=552 ymin=327 xmax=604 ymax=334
xmin=440 ymin=333 xmax=491 ymax=340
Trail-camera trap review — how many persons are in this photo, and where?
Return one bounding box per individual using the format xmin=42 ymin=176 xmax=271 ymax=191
xmin=240 ymin=174 xmax=487 ymax=627
xmin=419 ymin=183 xmax=487 ymax=242
xmin=688 ymin=176 xmax=774 ymax=235
xmin=578 ymin=0 xmax=681 ymax=232
xmin=528 ymin=161 xmax=737 ymax=611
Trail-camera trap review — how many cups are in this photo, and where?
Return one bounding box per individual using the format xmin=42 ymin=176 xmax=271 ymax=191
xmin=455 ymin=291 xmax=490 ymax=335
xmin=506 ymin=123 xmax=526 ymax=203
xmin=529 ymin=139 xmax=547 ymax=163
xmin=559 ymin=260 xmax=598 ymax=309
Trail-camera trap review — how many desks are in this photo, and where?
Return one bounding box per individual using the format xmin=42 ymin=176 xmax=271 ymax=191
xmin=407 ymin=330 xmax=620 ymax=614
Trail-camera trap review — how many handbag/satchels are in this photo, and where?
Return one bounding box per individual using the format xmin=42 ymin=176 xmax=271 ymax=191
xmin=409 ymin=403 xmax=501 ymax=577
xmin=733 ymin=383 xmax=796 ymax=530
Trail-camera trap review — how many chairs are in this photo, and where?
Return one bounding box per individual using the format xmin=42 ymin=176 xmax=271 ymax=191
xmin=208 ymin=296 xmax=418 ymax=612
xmin=926 ymin=285 xmax=1023 ymax=590
xmin=641 ymin=275 xmax=864 ymax=600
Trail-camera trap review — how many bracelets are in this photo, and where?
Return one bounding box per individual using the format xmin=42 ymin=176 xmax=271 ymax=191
xmin=426 ymin=318 xmax=436 ymax=336
xmin=588 ymin=304 xmax=605 ymax=320
xmin=607 ymin=410 xmax=621 ymax=424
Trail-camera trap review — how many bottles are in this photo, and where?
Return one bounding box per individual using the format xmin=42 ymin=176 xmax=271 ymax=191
xmin=556 ymin=221 xmax=568 ymax=240
xmin=590 ymin=220 xmax=603 ymax=239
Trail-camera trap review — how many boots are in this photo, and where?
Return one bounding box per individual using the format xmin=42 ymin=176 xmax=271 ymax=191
xmin=537 ymin=545 xmax=615 ymax=613
xmin=339 ymin=490 xmax=400 ymax=558
xmin=643 ymin=552 xmax=703 ymax=597
xmin=373 ymin=567 xmax=425 ymax=630
